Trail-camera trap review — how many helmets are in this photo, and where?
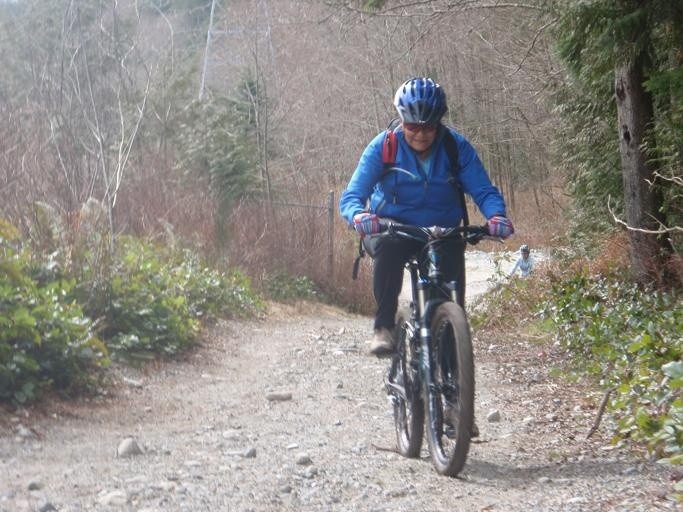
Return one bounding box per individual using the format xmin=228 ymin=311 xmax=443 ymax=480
xmin=392 ymin=75 xmax=450 ymax=127
xmin=520 ymin=244 xmax=530 ymax=254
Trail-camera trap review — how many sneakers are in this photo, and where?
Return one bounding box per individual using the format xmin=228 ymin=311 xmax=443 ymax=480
xmin=445 ymin=402 xmax=485 ymax=443
xmin=369 ymin=328 xmax=398 ymax=356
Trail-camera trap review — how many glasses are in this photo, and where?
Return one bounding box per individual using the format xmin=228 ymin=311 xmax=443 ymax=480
xmin=403 ymin=122 xmax=438 ymax=132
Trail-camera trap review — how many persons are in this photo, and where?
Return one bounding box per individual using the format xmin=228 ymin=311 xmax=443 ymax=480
xmin=503 ymin=244 xmax=536 ymax=283
xmin=337 ymin=73 xmax=516 ymax=438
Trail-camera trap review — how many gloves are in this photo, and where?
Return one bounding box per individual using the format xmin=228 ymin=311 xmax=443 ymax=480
xmin=485 ymin=215 xmax=515 ymax=239
xmin=352 ymin=212 xmax=383 ymax=236
